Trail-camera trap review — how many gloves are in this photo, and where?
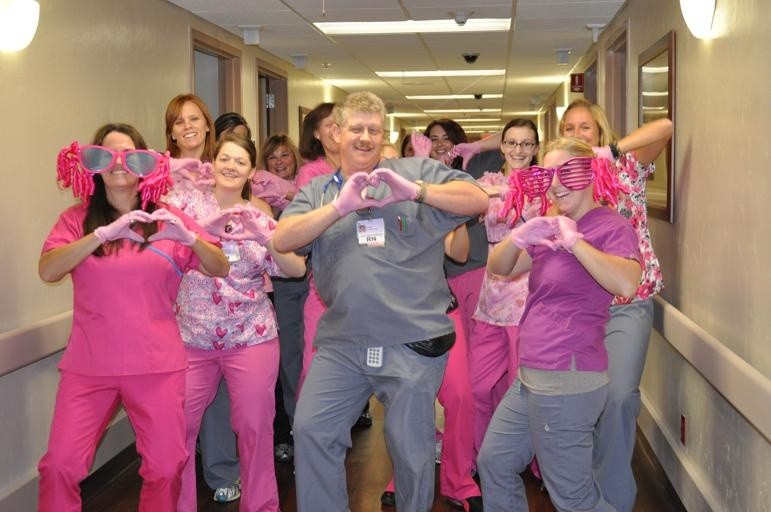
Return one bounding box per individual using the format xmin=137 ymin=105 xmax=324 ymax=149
xmin=257 ymin=176 xmax=289 ymax=202
xmin=451 ymin=140 xmax=481 ymax=169
xmin=510 ymin=217 xmax=552 ymax=250
xmin=95 ymin=211 xmax=151 ymax=243
xmin=200 ymin=164 xmax=219 ymax=187
xmin=374 ymin=167 xmax=419 ymax=209
xmin=147 ymin=209 xmax=196 ymax=246
xmin=410 ymin=130 xmax=432 ymax=159
xmin=334 ymin=171 xmax=374 ymax=219
xmin=552 ymin=217 xmax=585 ymax=252
xmin=170 ymin=156 xmax=201 ymax=184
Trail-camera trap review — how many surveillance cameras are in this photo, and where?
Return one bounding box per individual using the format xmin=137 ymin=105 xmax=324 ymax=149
xmin=449 ymin=10 xmax=474 ymax=26
xmin=471 ymin=91 xmax=483 ymax=100
xmin=461 ymin=52 xmax=480 ymax=64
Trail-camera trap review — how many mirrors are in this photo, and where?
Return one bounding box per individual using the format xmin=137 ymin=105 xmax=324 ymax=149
xmin=638 ymin=31 xmax=675 ymax=222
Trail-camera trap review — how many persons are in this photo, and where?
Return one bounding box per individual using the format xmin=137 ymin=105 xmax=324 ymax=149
xmin=40 ymin=93 xmax=675 ymax=511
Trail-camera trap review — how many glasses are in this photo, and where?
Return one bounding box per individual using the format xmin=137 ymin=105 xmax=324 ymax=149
xmin=57 ymin=142 xmax=174 ymax=210
xmin=500 ymin=156 xmax=631 ymax=225
xmin=504 ymin=138 xmax=537 ymax=150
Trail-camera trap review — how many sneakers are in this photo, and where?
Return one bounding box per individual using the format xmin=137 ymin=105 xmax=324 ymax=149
xmin=214 ymin=476 xmax=241 ymax=502
xmin=274 ymin=443 xmax=294 ymax=463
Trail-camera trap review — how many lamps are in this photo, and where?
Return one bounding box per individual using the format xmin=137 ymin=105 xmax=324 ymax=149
xmin=1 ymin=0 xmax=40 ymax=54
xmin=679 ymin=1 xmax=717 ymax=39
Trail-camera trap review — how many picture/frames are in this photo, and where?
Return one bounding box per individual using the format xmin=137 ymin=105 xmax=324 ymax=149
xmin=298 ymin=106 xmax=309 ymax=132
